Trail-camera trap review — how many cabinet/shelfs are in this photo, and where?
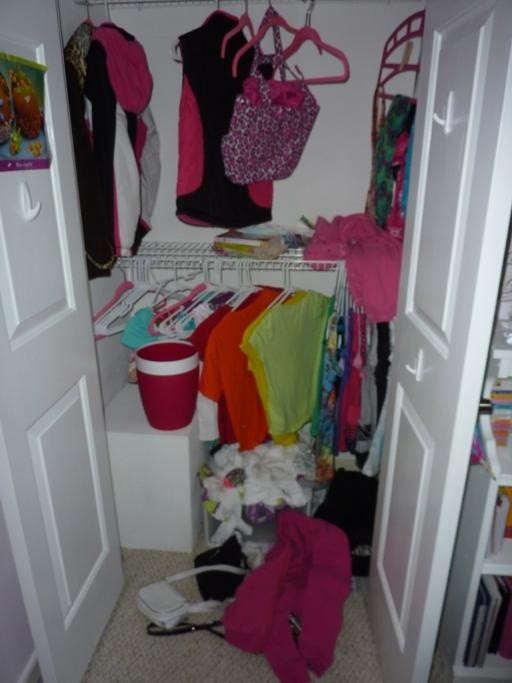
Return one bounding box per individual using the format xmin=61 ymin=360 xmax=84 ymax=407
xmin=442 ymin=404 xmax=512 ymax=682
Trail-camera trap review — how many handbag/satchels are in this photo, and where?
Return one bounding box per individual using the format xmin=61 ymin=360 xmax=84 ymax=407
xmin=135 ymin=581 xmax=190 ymax=630
xmin=194 ymin=534 xmax=247 ymax=601
xmin=223 ymin=5 xmax=319 ymax=183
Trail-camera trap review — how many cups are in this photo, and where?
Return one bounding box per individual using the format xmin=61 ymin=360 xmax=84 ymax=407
xmin=134 ymin=340 xmax=200 ymax=431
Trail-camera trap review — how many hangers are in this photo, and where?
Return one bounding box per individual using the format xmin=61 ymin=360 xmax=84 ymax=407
xmin=67 ymin=1 xmax=354 ymax=91
xmin=88 ymin=250 xmax=375 ymax=367
